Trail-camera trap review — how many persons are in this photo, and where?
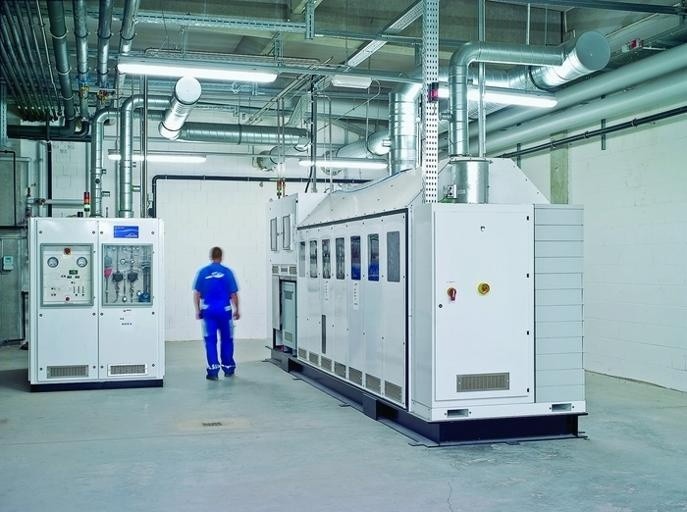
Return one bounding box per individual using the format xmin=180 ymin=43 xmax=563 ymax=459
xmin=369 ymin=255 xmax=379 ymax=281
xmin=192 ymin=247 xmax=241 ymax=380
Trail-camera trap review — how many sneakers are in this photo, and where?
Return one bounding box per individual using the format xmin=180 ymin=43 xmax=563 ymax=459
xmin=206 ymin=371 xmax=234 ymax=380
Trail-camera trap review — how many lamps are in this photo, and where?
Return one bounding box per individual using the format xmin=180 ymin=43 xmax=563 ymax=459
xmin=114 ymin=56 xmax=278 ymax=86
xmin=107 ymin=147 xmax=207 ymax=165
xmin=431 ymin=81 xmax=560 ymax=111
xmin=297 ymin=157 xmax=388 ymax=171
